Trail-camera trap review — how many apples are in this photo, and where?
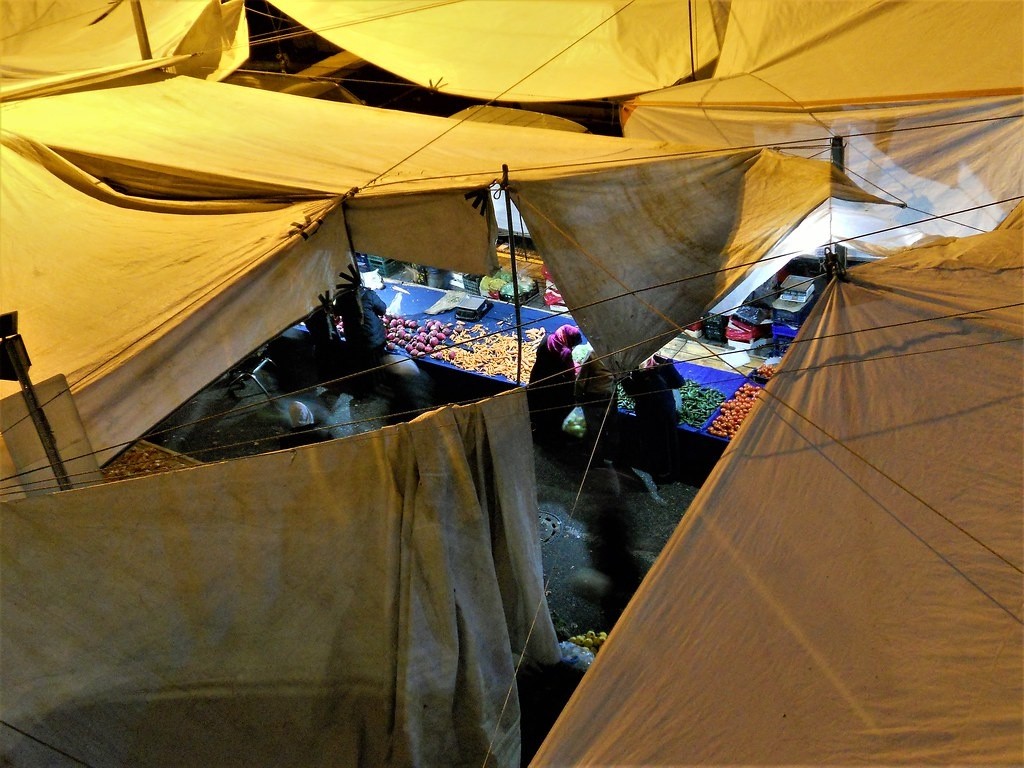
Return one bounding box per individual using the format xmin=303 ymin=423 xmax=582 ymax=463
xmin=567 ymin=630 xmax=607 ymax=655
xmin=756 ymin=363 xmax=778 ymax=378
xmin=708 ymin=383 xmax=762 ymax=439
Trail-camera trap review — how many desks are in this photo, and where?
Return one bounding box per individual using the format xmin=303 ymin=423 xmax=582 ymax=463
xmin=293 ymin=278 xmax=588 ymax=388
xmin=617 ymin=360 xmax=767 ymax=442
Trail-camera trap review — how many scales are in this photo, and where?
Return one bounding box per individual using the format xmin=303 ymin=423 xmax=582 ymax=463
xmin=780 ymin=274 xmax=815 ymax=303
xmin=455 ymin=294 xmax=492 ymax=322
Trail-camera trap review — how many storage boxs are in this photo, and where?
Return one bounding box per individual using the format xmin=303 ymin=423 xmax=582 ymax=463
xmin=705 ymin=315 xmax=759 ymax=350
xmin=498 ymin=280 xmax=539 ymax=304
xmin=367 ymin=254 xmax=408 ymax=278
xmin=771 ymin=295 xmax=816 ymax=358
xmin=462 ymin=273 xmax=485 ymax=294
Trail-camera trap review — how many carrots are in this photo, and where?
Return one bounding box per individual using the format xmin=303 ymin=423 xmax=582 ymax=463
xmin=430 ymin=322 xmax=547 ymax=385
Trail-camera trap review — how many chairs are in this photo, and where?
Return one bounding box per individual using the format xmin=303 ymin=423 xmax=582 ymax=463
xmin=229 ymin=357 xmax=280 ymax=397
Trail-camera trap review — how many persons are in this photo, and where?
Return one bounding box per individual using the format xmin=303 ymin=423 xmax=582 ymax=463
xmin=528 ymin=324 xmax=583 ymax=451
xmin=279 ymin=401 xmax=335 ymax=451
xmin=573 ymin=346 xmax=686 ymax=478
xmin=304 ymin=284 xmax=387 ymax=390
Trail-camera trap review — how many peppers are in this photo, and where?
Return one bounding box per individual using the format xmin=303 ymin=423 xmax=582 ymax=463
xmin=615 ymin=380 xmax=726 ymax=428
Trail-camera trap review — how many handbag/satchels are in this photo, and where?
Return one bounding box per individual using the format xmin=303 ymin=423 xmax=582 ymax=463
xmin=562 ymin=407 xmax=586 ymax=438
